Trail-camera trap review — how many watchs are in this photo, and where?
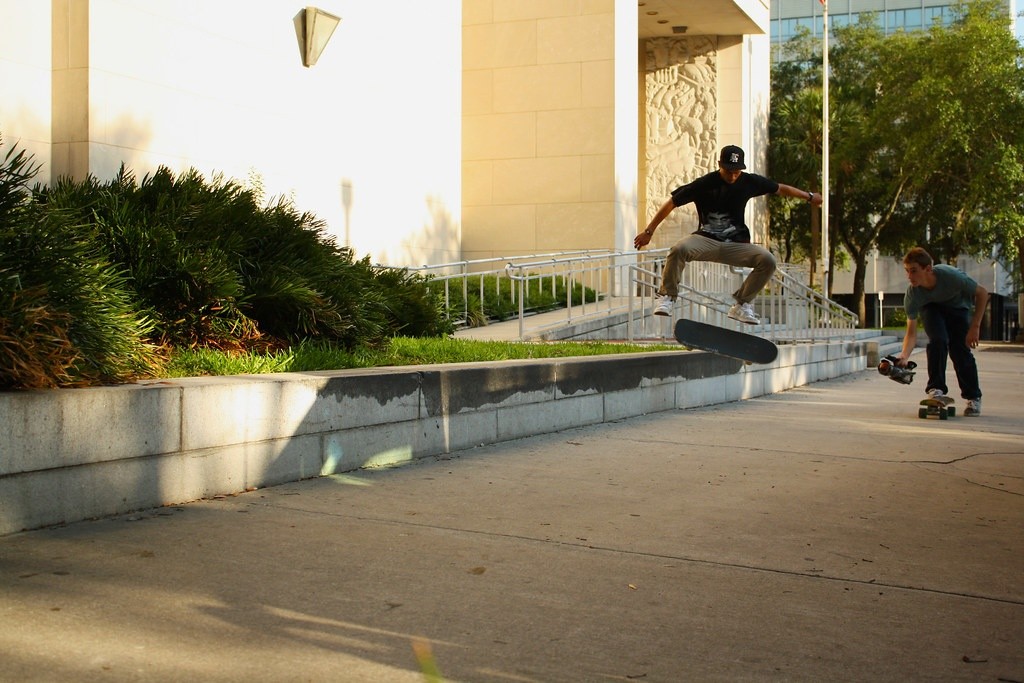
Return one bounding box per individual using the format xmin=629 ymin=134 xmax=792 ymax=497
xmin=808 ymin=191 xmax=813 ymax=201
xmin=644 ymin=228 xmax=653 ymax=236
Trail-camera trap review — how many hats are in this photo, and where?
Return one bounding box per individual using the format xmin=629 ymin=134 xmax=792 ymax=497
xmin=720 ymin=145 xmax=746 ymax=172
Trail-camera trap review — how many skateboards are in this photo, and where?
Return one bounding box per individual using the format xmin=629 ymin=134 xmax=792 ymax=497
xmin=673 ymin=319 xmax=779 ymax=365
xmin=918 ymin=396 xmax=956 ymax=419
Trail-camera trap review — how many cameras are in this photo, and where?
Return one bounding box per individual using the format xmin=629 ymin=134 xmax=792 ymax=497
xmin=878 ymin=354 xmax=917 ymax=386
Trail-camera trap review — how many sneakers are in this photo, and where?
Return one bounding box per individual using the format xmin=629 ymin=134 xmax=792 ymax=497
xmin=964 ymin=397 xmax=982 ymax=417
xmin=653 ymin=295 xmax=675 ymax=317
xmin=927 ymin=389 xmax=954 ymax=406
xmin=727 ymin=301 xmax=760 ymax=325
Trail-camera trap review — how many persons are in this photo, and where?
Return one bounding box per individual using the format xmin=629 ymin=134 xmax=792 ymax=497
xmin=633 ymin=145 xmax=822 ymax=325
xmin=702 ymin=203 xmax=735 ymax=234
xmin=893 ymin=247 xmax=988 ymax=417
